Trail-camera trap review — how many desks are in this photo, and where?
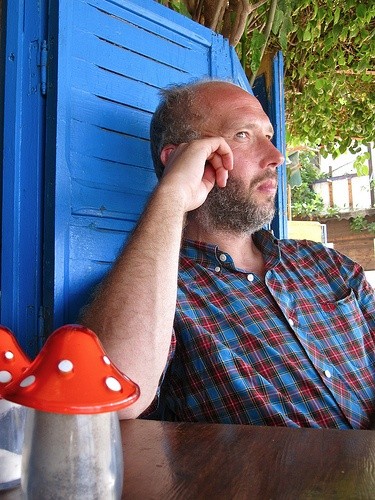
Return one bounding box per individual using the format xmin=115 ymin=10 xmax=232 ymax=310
xmin=0 ymin=417 xmax=375 ymax=500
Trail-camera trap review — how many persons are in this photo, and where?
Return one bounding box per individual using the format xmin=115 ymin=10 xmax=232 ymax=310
xmin=78 ymin=77 xmax=375 ymax=430
